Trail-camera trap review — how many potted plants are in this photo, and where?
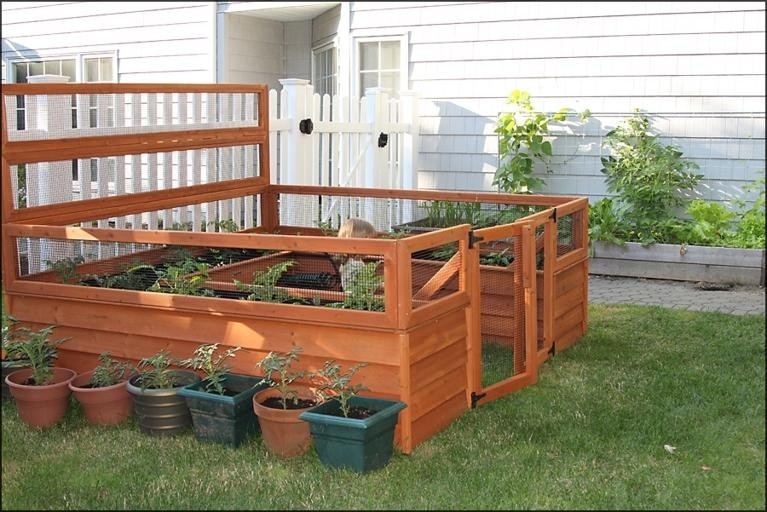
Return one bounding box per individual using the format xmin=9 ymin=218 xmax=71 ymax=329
xmin=1 ymin=315 xmax=52 ymax=391
xmin=70 ymin=352 xmax=139 ymax=428
xmin=178 ymin=343 xmax=269 ymax=449
xmin=303 ymin=360 xmax=406 ymax=473
xmin=127 ymin=347 xmax=202 ymax=438
xmin=3 ymin=326 xmax=77 ymax=427
xmin=390 ymin=199 xmax=544 ymax=257
xmin=252 ymin=348 xmax=325 ymax=458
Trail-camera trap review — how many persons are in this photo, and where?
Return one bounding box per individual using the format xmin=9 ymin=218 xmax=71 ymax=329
xmin=332 ymin=218 xmax=384 ymax=295
